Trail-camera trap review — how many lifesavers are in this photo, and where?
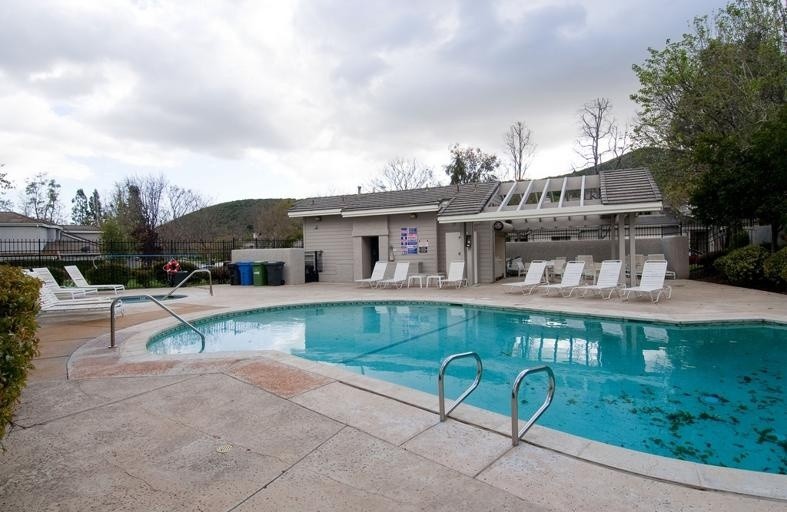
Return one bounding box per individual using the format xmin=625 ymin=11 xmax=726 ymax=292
xmin=167 ymin=260 xmax=180 ymax=272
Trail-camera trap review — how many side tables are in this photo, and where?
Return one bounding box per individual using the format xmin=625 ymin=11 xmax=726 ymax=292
xmin=408 ymin=275 xmax=427 ymax=289
xmin=426 ymin=275 xmax=446 ymax=289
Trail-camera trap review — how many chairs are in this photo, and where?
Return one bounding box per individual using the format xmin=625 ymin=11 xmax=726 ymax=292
xmin=375 ymin=262 xmax=410 ymax=288
xmin=440 ymin=262 xmax=472 ymax=289
xmin=501 ymin=254 xmax=676 ymax=303
xmin=21 ymin=265 xmax=125 ymax=320
xmin=354 ymin=261 xmax=389 ymax=290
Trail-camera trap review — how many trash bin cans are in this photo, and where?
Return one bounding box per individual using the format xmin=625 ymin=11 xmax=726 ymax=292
xmin=228 ymin=261 xmax=285 ymax=286
